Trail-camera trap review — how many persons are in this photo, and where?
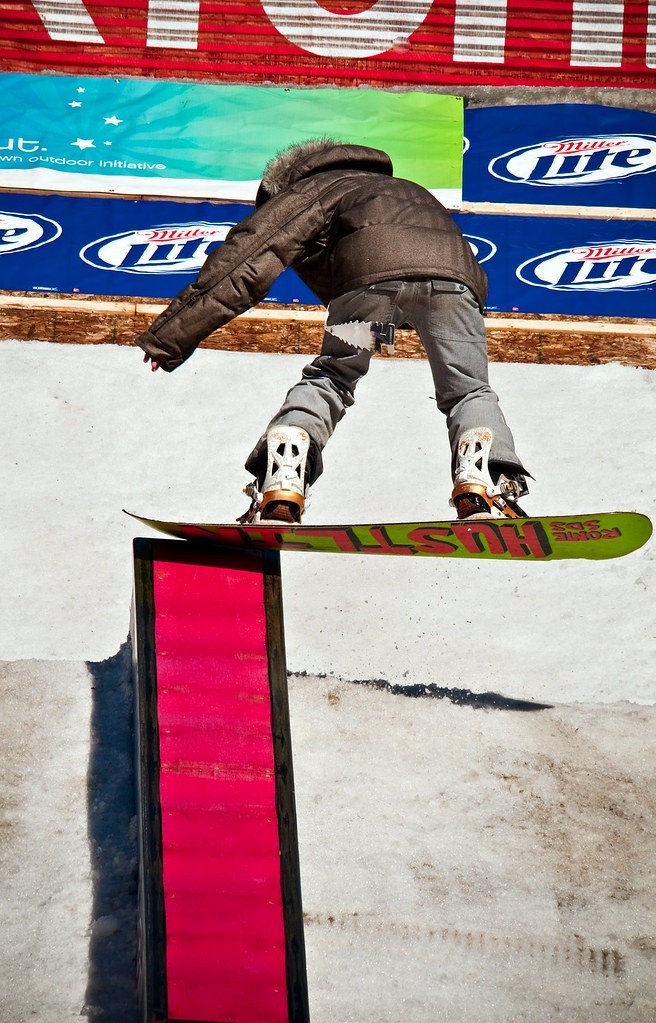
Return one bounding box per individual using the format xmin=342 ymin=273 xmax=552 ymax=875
xmin=134 ymin=139 xmax=535 ymax=524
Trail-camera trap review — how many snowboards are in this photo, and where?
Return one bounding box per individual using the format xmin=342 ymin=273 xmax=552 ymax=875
xmin=122 ymin=508 xmax=653 ymax=560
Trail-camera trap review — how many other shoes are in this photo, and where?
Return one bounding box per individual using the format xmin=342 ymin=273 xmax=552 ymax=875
xmin=447 ymin=461 xmax=531 ymax=521
xmin=236 ymin=444 xmax=313 ymax=524
